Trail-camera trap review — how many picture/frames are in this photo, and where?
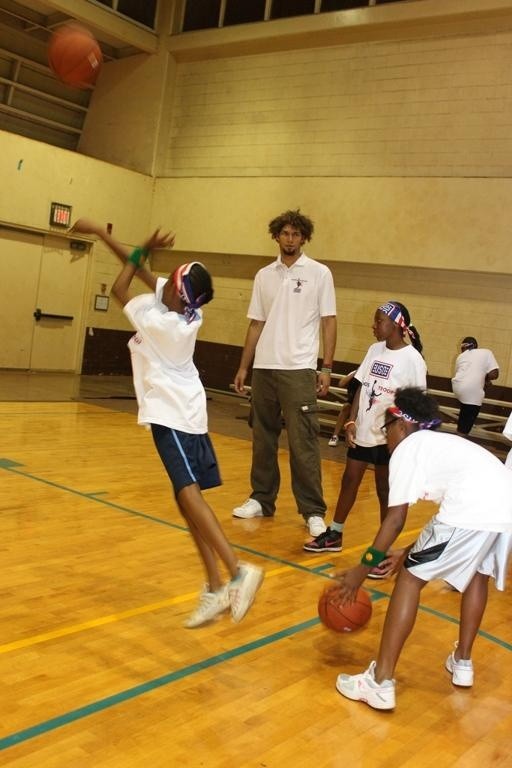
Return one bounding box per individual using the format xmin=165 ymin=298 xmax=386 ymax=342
xmin=94 ymin=294 xmax=110 ymax=311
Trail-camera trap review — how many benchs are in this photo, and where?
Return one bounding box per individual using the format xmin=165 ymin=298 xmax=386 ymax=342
xmin=229 ymin=362 xmax=512 ymax=462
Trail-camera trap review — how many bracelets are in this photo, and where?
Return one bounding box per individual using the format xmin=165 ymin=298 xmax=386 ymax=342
xmin=360 ymin=547 xmax=385 ymax=567
xmin=321 ymin=368 xmax=332 ymax=373
xmin=343 ymin=421 xmax=355 ymax=430
xmin=127 ymin=246 xmax=149 ymax=267
xmin=321 ymin=364 xmax=333 ymax=368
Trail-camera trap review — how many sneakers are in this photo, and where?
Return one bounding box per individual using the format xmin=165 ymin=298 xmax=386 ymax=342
xmin=231 ymin=499 xmax=265 ymax=519
xmin=228 ymin=560 xmax=264 ymax=623
xmin=446 ymin=652 xmax=474 ymax=687
xmin=306 ymin=516 xmax=326 ymax=537
xmin=304 ymin=527 xmax=342 ymax=552
xmin=368 ymin=558 xmax=391 ymax=579
xmin=329 ymin=435 xmax=339 ymax=446
xmin=185 ymin=581 xmax=232 ymax=629
xmin=335 ymin=662 xmax=396 ymax=710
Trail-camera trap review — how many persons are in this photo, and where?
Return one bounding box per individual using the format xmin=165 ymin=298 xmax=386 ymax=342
xmin=67 ymin=217 xmax=265 ymax=628
xmin=451 ymin=337 xmax=499 ymax=438
xmin=328 ymin=366 xmax=361 ymax=447
xmin=231 ymin=208 xmax=337 ymax=537
xmin=329 ymin=385 xmax=512 ymax=710
xmin=303 ymin=301 xmax=428 ymax=581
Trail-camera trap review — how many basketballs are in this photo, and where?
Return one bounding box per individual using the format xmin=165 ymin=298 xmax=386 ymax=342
xmin=49 ymin=30 xmax=103 ymax=89
xmin=319 ymin=582 xmax=372 ymax=633
